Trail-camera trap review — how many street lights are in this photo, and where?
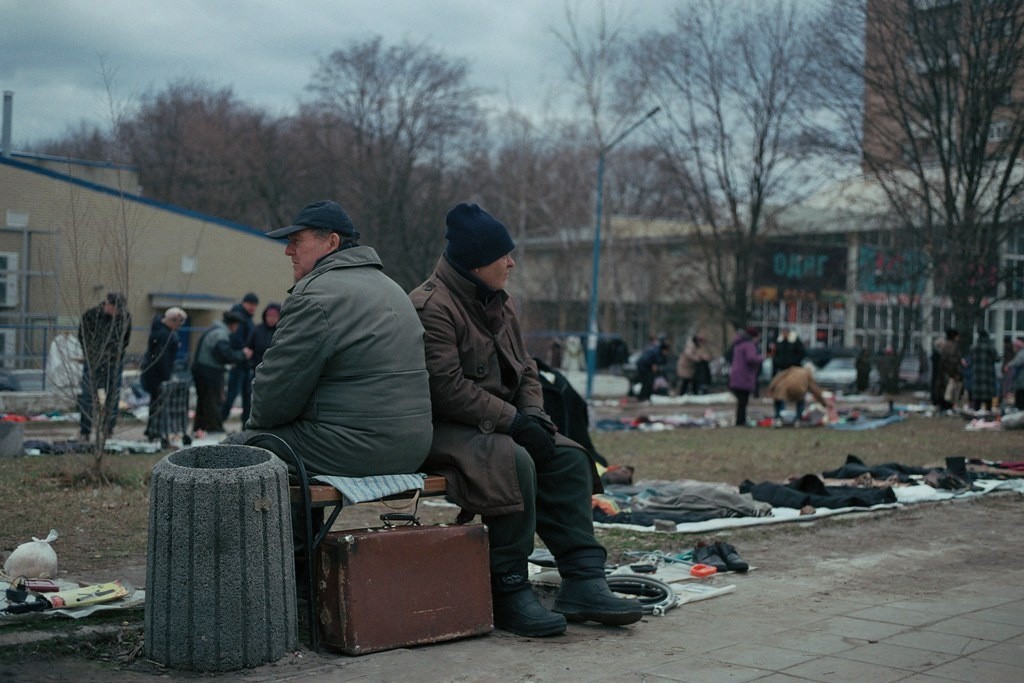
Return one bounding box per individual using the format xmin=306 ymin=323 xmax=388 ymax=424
xmin=585 ymin=106 xmax=661 ymax=400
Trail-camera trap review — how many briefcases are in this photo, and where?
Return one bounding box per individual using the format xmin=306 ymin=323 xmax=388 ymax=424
xmin=314 ymin=513 xmax=495 ymax=655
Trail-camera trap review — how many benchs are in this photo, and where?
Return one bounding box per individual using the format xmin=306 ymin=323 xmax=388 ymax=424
xmin=242 ymin=433 xmax=446 ymax=654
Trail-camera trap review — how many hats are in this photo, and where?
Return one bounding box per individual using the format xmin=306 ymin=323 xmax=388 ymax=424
xmin=978 ymin=328 xmax=989 ymax=339
xmin=445 ymin=203 xmax=515 ymax=270
xmin=243 ymin=294 xmax=258 ymax=304
xmin=223 ymin=312 xmax=245 ymax=324
xmin=266 ymin=200 xmax=360 ymax=240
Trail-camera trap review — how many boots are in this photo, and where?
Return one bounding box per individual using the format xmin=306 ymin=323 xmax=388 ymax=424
xmin=946 ymin=457 xmax=986 ymax=491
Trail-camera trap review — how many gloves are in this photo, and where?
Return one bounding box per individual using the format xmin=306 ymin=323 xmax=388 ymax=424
xmin=508 ymin=413 xmax=556 ymax=461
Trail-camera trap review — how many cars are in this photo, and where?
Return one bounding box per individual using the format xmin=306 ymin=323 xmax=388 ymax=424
xmin=816 ymin=357 xmax=883 ymax=396
xmin=892 ymin=356 xmax=934 ymax=391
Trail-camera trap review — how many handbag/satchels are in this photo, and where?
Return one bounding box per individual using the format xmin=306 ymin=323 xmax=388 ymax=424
xmin=723 ymin=344 xmax=734 ymax=362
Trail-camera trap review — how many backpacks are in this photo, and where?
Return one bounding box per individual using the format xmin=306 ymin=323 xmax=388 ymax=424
xmin=530 ymin=356 xmax=608 ymax=468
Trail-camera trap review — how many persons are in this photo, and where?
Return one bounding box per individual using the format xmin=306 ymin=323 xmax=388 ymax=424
xmin=45 ymin=326 xmax=84 ymax=391
xmin=855 ymin=346 xmax=901 ymax=395
xmin=78 ymin=293 xmax=132 ymax=441
xmin=217 ymin=202 xmax=434 ymax=612
xmin=406 ymin=203 xmax=642 ymax=637
xmin=917 ymin=325 xmax=1024 ymax=423
xmin=139 ymin=306 xmax=192 ymax=450
xmin=190 ymin=292 xmax=282 ymax=439
xmin=628 ymin=325 xmax=833 ymax=430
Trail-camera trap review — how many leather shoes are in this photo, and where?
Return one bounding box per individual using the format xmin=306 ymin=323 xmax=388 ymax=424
xmin=693 ymin=540 xmax=748 ymax=572
xmin=551 ymin=577 xmax=643 ymax=625
xmin=493 ymin=589 xmax=567 ymax=637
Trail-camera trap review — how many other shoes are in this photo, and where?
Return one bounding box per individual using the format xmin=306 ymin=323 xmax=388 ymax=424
xmin=775 ymin=417 xmax=783 ymax=428
xmin=794 ymin=418 xmax=802 ymax=428
